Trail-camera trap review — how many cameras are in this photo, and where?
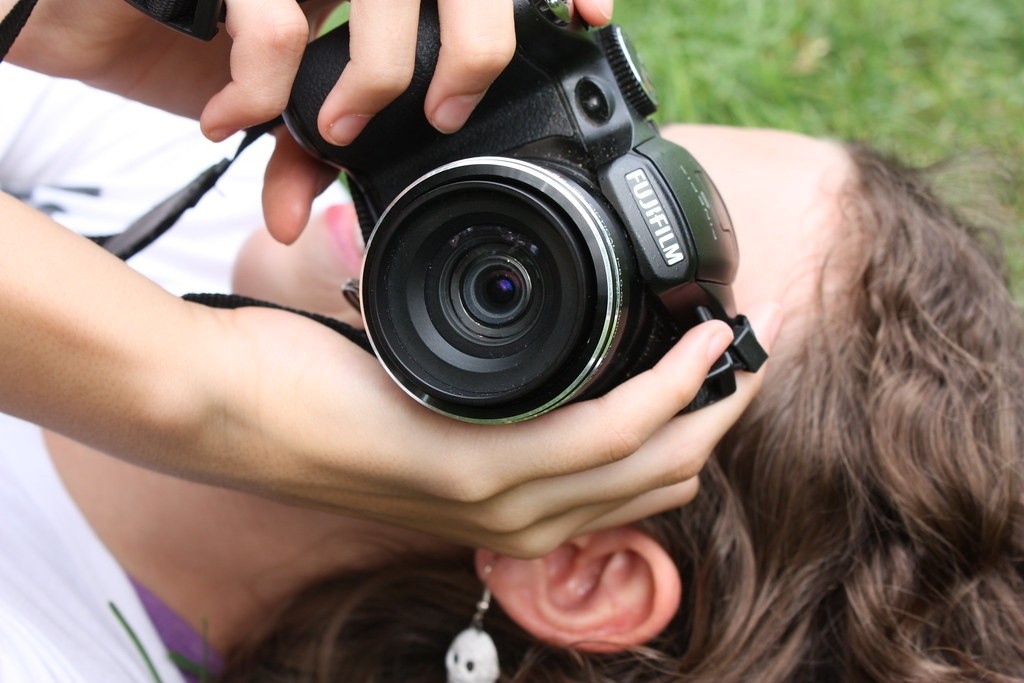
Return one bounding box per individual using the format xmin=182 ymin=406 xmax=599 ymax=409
xmin=275 ymin=0 xmax=773 ymax=425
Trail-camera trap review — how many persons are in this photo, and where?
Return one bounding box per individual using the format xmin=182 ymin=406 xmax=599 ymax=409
xmin=1 ymin=1 xmax=1024 ymax=683
